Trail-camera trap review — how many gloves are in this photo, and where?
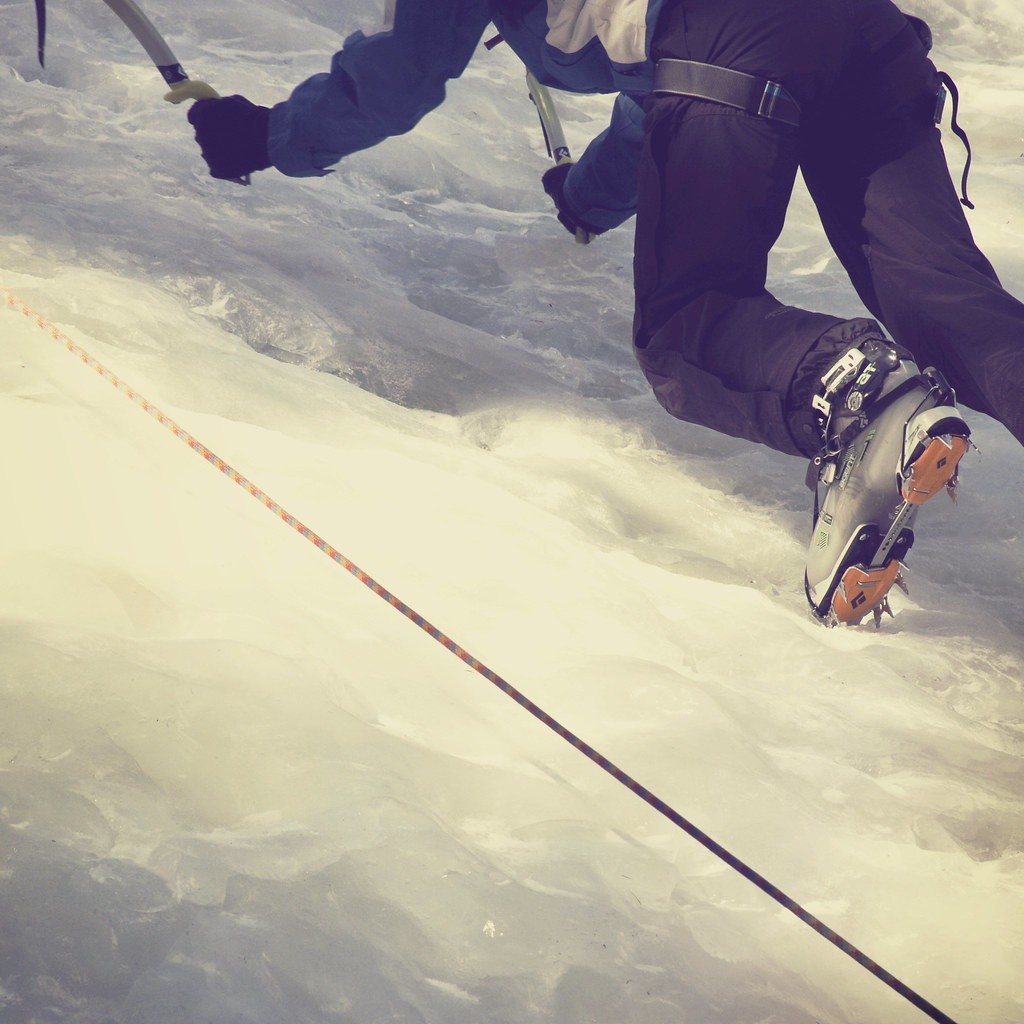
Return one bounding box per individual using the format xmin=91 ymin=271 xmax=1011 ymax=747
xmin=543 ymin=165 xmax=608 ymax=238
xmin=187 ymin=94 xmax=274 ymax=179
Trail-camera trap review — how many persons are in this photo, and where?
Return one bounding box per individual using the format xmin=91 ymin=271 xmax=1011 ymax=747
xmin=187 ymin=0 xmax=1024 ymax=631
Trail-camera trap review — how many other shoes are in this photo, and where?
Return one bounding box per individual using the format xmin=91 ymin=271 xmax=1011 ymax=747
xmin=805 ymin=349 xmax=967 ymax=628
